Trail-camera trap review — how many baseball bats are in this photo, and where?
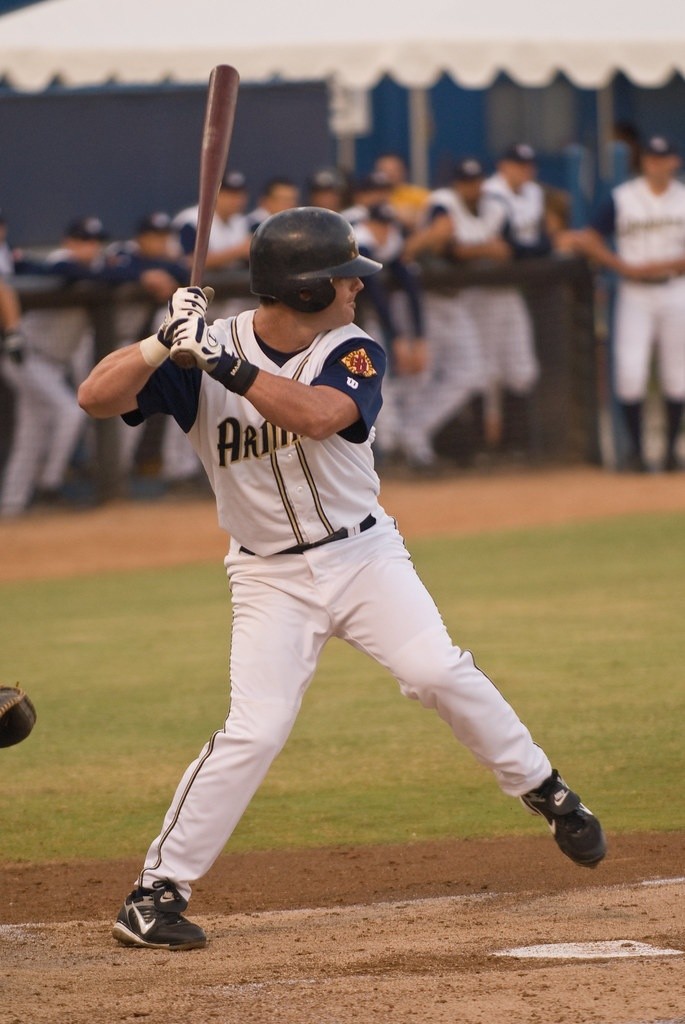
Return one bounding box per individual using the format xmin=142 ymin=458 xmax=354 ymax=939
xmin=172 ymin=63 xmax=241 ymax=370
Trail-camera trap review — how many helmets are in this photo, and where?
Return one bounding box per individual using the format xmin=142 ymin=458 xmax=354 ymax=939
xmin=506 ymin=143 xmax=536 ymax=161
xmin=248 ymin=206 xmax=384 ymax=312
xmin=456 ymin=159 xmax=483 ymax=179
xmin=137 ymin=210 xmax=177 ymax=234
xmin=221 ymin=169 xmax=249 ymax=191
xmin=639 ymin=130 xmax=681 ymax=154
xmin=63 ymin=216 xmax=108 ymax=241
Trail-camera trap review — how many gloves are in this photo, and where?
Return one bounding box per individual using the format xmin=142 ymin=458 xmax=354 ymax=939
xmin=4 ymin=326 xmax=28 ymax=367
xmin=161 ymin=316 xmax=259 ymax=396
xmin=139 ymin=286 xmax=215 ymax=370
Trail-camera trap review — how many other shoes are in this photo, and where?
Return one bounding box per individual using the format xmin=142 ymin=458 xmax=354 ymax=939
xmin=0 ymin=686 xmax=37 ymax=747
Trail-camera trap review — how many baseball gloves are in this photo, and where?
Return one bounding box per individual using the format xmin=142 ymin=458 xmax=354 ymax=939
xmin=0 ymin=678 xmax=38 ymax=749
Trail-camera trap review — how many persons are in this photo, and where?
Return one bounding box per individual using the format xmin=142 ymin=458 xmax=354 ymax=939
xmin=0 ymin=134 xmax=685 ymax=498
xmin=79 ymin=204 xmax=608 ymax=954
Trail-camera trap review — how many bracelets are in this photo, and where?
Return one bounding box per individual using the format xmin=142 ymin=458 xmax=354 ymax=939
xmin=222 ymin=357 xmax=260 ymax=397
xmin=140 ymin=333 xmax=171 ymax=366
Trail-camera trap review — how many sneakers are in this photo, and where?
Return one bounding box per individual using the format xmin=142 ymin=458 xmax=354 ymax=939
xmin=518 ymin=768 xmax=607 ymax=868
xmin=110 ymin=876 xmax=207 ymax=949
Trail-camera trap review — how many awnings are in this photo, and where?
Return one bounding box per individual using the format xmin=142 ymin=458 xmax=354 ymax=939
xmin=0 ymin=0 xmax=685 ymax=102
